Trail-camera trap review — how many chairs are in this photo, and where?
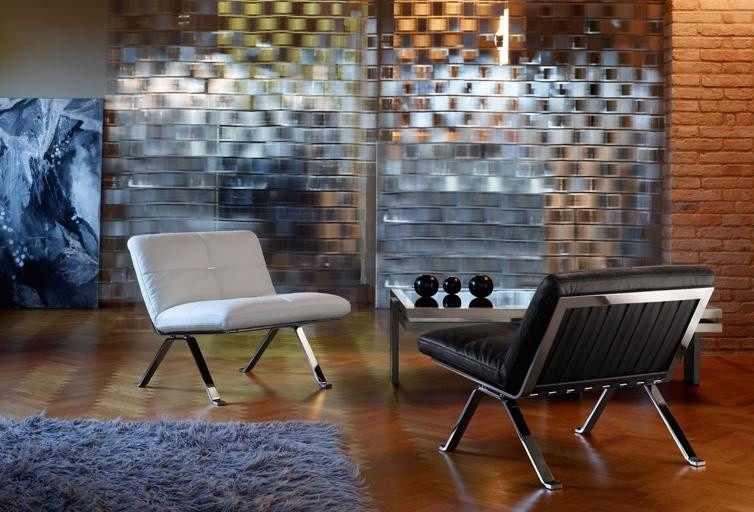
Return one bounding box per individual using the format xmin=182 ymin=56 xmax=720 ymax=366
xmin=416 ymin=265 xmax=716 ymax=490
xmin=127 ymin=230 xmax=351 ymax=406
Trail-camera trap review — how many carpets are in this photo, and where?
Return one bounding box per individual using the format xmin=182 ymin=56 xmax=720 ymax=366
xmin=0 ymin=416 xmax=373 ymax=511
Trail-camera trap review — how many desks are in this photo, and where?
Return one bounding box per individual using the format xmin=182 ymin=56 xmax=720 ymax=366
xmin=390 ymin=287 xmax=723 ymax=387
